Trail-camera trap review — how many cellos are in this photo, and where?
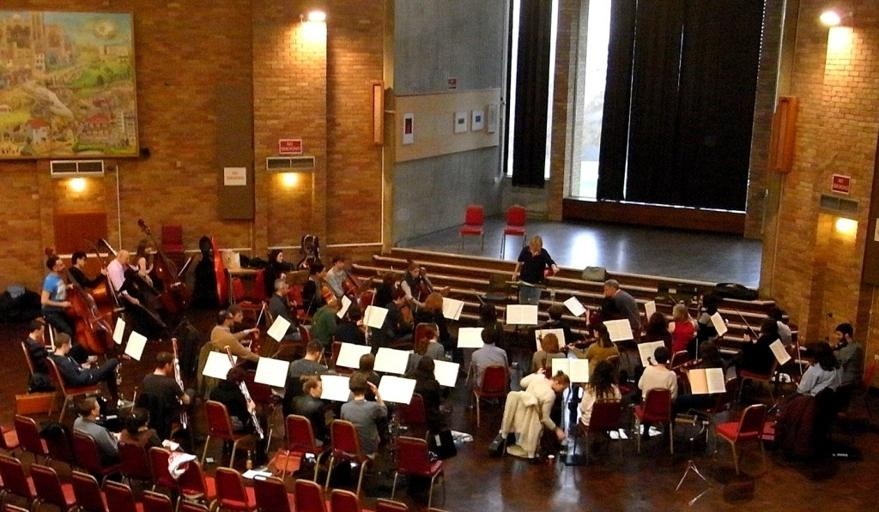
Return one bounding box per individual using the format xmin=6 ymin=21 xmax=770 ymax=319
xmin=46 ymin=245 xmax=115 ymax=353
xmin=138 ymin=218 xmax=192 ymax=316
xmin=342 ymin=265 xmax=362 ymax=299
xmin=302 ymin=262 xmax=340 ymax=309
xmin=406 ymin=256 xmax=433 ymax=303
xmin=90 ymin=244 xmax=118 ymax=331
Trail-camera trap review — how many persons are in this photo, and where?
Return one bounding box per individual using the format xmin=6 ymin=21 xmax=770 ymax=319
xmin=26 ymin=239 xmax=464 ymax=465
xmin=515 ymin=236 xmax=559 ymax=305
xmin=471 ymin=280 xmax=864 ymax=462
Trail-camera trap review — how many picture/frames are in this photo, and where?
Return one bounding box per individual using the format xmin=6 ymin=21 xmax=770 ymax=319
xmin=0 ymin=11 xmax=140 ymax=161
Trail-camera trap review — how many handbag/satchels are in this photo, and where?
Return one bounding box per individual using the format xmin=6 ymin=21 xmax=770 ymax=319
xmin=582 ymin=267 xmax=607 ymax=282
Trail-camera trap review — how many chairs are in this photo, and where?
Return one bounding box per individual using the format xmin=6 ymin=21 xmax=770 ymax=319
xmin=471 ymin=365 xmax=510 ymax=426
xmin=631 ymin=387 xmax=675 ymax=454
xmin=670 ymin=349 xmax=690 ymax=369
xmin=501 ymin=203 xmax=527 ymax=262
xmin=837 ymin=381 xmax=855 ymax=413
xmin=459 ymin=204 xmax=485 ymax=249
xmin=739 ymin=353 xmax=778 ymax=404
xmin=504 ymin=390 xmax=553 ymax=457
xmin=715 ymin=403 xmax=767 ymax=471
xmin=681 ymin=377 xmax=742 ymax=432
xmin=161 ymin=224 xmax=184 ymax=265
xmin=762 ymin=394 xmax=813 ymax=465
xmin=814 ymin=387 xmax=838 ymax=436
xmin=575 ymin=398 xmax=624 ymax=464
